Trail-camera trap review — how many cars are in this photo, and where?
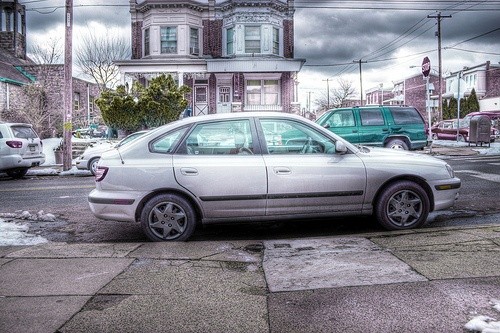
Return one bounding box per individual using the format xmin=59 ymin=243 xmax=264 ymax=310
xmin=88 ymin=111 xmax=462 ymax=242
xmin=428 ymin=118 xmax=500 ymax=143
xmin=75 ymin=129 xmax=153 ymax=176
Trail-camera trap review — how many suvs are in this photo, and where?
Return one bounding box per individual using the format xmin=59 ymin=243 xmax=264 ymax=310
xmin=0 ymin=120 xmax=46 ymax=179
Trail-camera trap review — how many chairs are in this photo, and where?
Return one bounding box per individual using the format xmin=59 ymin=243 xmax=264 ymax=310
xmin=186 ymin=130 xmax=254 ymax=155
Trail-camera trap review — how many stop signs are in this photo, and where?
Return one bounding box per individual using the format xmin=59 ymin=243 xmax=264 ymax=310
xmin=421 ymin=56 xmax=430 ymax=78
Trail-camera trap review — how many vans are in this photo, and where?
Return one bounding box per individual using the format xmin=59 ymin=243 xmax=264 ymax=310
xmin=273 ymin=103 xmax=430 ymax=170
